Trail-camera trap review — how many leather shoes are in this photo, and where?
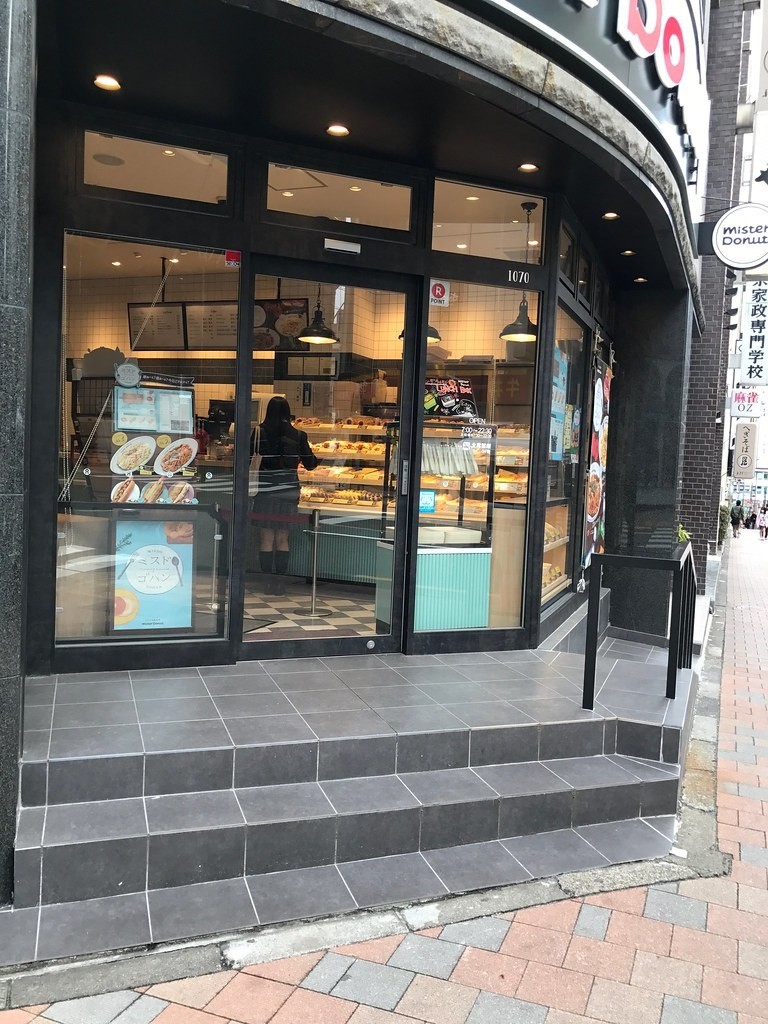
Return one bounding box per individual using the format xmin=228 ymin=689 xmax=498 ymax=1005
xmin=264 ymin=582 xmax=284 ymax=596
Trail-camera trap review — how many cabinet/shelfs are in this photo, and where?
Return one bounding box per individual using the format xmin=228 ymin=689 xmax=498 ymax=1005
xmin=375 ymin=420 xmax=498 ymax=632
xmin=487 ymin=495 xmax=572 ymax=627
xmin=253 ymin=422 xmax=533 ymax=588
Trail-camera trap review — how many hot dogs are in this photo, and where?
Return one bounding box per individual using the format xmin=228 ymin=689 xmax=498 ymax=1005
xmin=112 ymin=442 xmax=193 ymax=504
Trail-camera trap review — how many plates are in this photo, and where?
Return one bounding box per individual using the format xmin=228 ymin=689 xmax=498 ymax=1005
xmin=386 ymin=526 xmax=481 ymax=543
xmin=109 ymin=435 xmax=157 ymax=474
xmin=138 ymin=480 xmax=168 ymax=503
xmin=153 ymin=438 xmax=200 ymax=476
xmin=587 ymin=462 xmax=603 ymax=522
xmin=165 ymin=481 xmax=195 ymax=503
xmin=110 ymin=479 xmax=140 ymax=503
xmin=597 ymin=415 xmax=609 ymax=473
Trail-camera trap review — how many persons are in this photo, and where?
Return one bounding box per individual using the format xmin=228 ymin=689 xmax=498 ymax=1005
xmin=750 ymin=511 xmax=758 ymax=529
xmin=756 ymin=507 xmax=766 ymax=540
xmin=248 ymin=396 xmax=324 ymax=597
xmin=730 ymin=500 xmax=744 ymax=538
xmin=743 ymin=516 xmax=751 ymax=530
xmin=762 ymin=503 xmax=768 ymax=540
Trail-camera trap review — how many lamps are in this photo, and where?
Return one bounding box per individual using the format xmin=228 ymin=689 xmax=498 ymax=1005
xmin=399 ymin=324 xmax=441 ymax=343
xmin=297 ymin=284 xmax=340 ymax=345
xmin=498 ymin=202 xmax=539 ymax=343
